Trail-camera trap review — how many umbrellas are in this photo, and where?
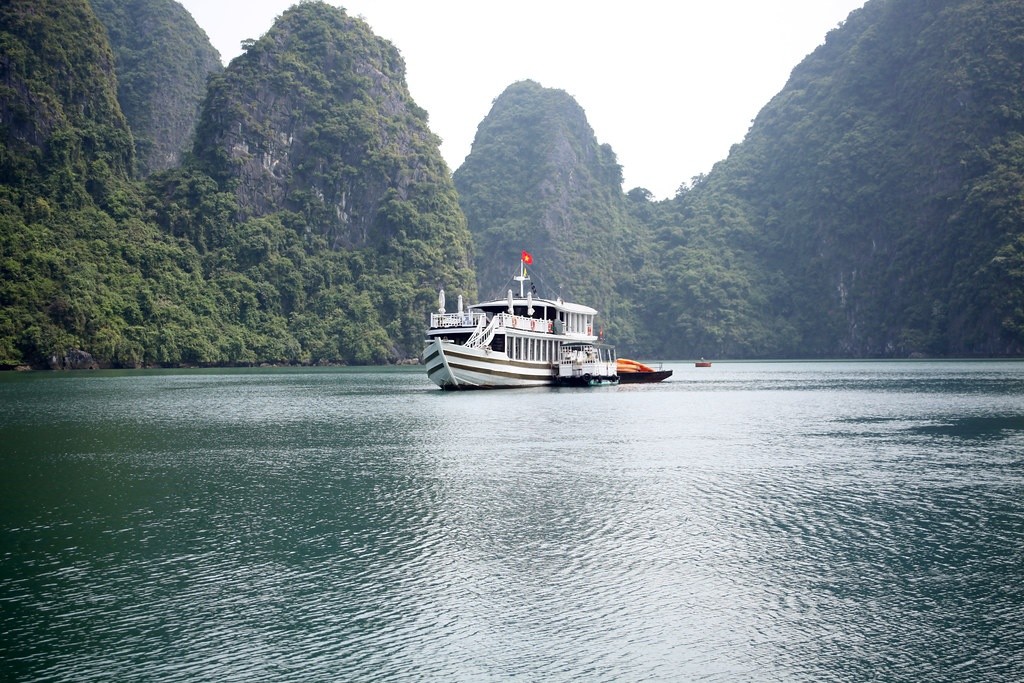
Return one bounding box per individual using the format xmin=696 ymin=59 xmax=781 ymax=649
xmin=457 ymin=294 xmax=464 ymax=317
xmin=508 ymin=289 xmax=514 ymax=315
xmin=527 ymin=292 xmax=535 ymax=318
xmin=437 ymin=290 xmax=446 ymax=314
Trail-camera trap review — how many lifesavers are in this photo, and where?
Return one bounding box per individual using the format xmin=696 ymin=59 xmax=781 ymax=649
xmin=583 ymin=373 xmax=592 ymax=382
xmin=512 ymin=317 xmax=518 ymax=326
xmin=547 ymin=323 xmax=554 ymax=334
xmin=530 ymin=321 xmax=535 ymax=329
xmin=588 ymin=326 xmax=591 ymax=335
xmin=599 ymin=330 xmax=603 ymax=340
xmin=597 ymin=374 xmax=602 ymax=384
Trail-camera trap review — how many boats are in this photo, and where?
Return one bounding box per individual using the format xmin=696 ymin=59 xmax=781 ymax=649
xmin=616 ymin=358 xmax=674 ymax=383
xmin=552 ymin=342 xmax=620 ymax=385
xmin=695 ymin=361 xmax=713 ymax=367
xmin=422 ymin=250 xmax=601 ymax=389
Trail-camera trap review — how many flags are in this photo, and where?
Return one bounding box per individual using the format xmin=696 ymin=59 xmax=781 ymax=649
xmin=521 ymin=249 xmax=533 ymax=265
